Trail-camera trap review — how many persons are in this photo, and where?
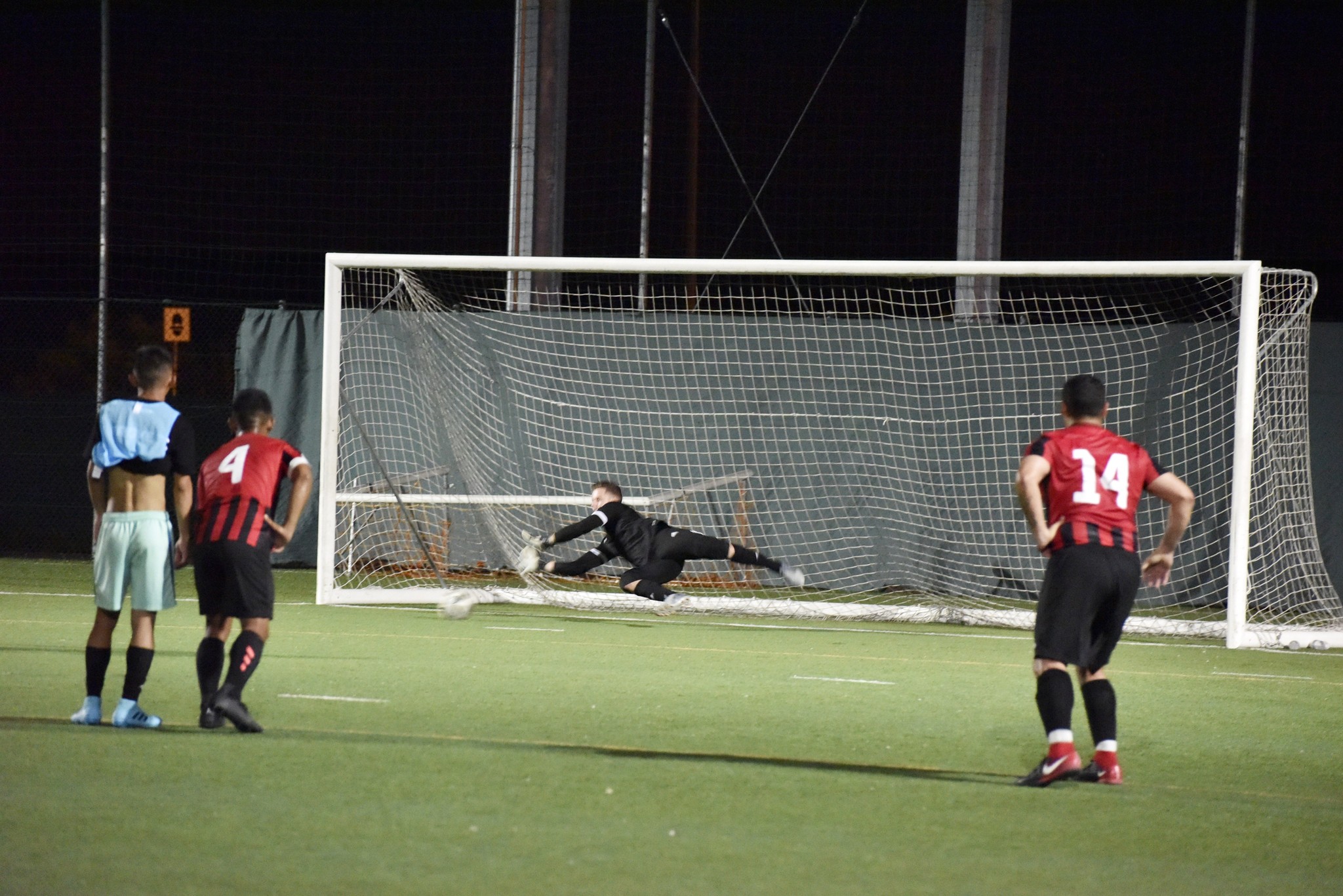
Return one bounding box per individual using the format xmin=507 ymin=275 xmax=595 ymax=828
xmin=70 ymin=344 xmax=192 ymax=731
xmin=193 ymin=389 xmax=313 ymax=732
xmin=518 ymin=481 xmax=805 ymax=617
xmin=1015 ymin=374 xmax=1196 ymax=788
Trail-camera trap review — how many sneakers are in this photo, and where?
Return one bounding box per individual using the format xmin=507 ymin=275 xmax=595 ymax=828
xmin=70 ymin=708 xmax=103 ymax=725
xmin=200 ymin=709 xmax=225 ymax=728
xmin=1066 ymin=762 xmax=1124 ymax=785
xmin=112 ymin=706 xmax=162 ymax=728
xmin=1017 ymin=752 xmax=1080 ymax=791
xmin=213 ymin=690 xmax=263 ymax=733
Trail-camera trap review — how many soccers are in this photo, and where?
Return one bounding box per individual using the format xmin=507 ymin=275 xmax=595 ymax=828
xmin=435 ymin=587 xmax=475 ymax=620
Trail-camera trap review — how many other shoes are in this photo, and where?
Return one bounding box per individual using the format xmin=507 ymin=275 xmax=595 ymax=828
xmin=780 ymin=559 xmax=805 ymax=590
xmin=653 ymin=592 xmax=693 ymax=615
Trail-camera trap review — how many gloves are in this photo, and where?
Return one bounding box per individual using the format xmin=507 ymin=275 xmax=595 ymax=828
xmin=517 ymin=529 xmax=552 ymax=577
